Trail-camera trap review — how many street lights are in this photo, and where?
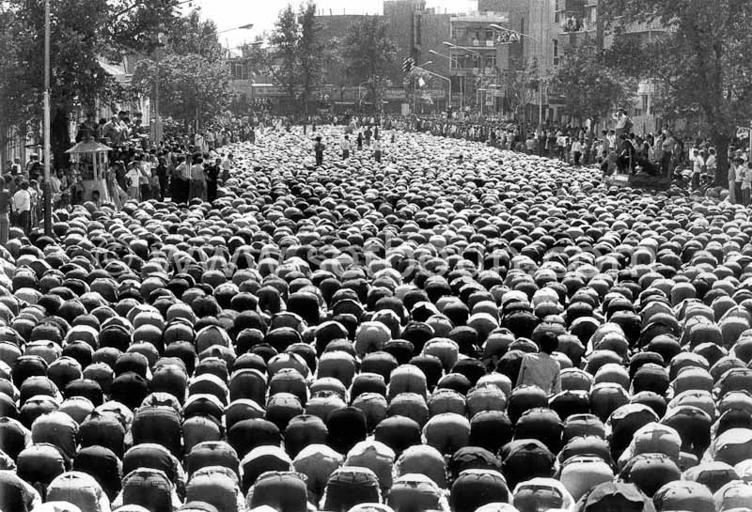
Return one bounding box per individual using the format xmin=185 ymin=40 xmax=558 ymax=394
xmin=427 ymin=46 xmax=462 ymax=110
xmin=221 ymin=40 xmax=264 ymax=52
xmin=486 ymin=21 xmax=544 ymax=129
xmin=411 ymin=64 xmax=452 ymax=111
xmin=438 ymin=39 xmax=487 ymax=113
xmin=196 ymin=21 xmax=256 ymax=125
xmin=408 ymin=59 xmax=433 ymax=74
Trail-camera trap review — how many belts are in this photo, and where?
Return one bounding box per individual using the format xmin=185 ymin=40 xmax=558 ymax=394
xmin=66 ymin=397 xmax=88 ymax=401
xmin=188 ymin=471 xmax=229 ymax=477
xmin=394 ymin=480 xmax=431 ymax=487
xmin=568 ymin=415 xmax=600 ymax=422
xmin=569 ymin=458 xmax=607 ymax=464
xmin=518 ymin=483 xmax=559 ymax=493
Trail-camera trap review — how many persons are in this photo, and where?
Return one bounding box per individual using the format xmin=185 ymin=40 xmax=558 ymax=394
xmin=1 ymin=111 xmax=752 ymax=512
